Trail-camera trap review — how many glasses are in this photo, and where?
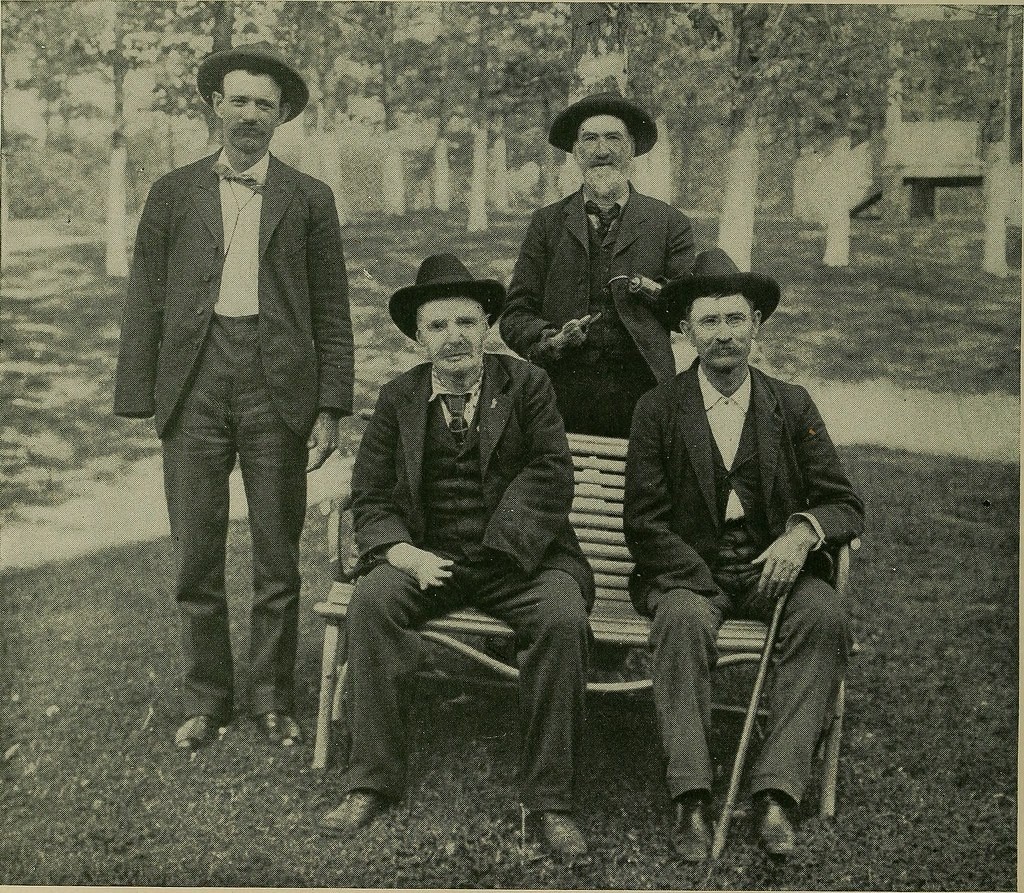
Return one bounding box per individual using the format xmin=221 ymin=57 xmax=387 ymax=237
xmin=696 ymin=314 xmax=747 ymax=331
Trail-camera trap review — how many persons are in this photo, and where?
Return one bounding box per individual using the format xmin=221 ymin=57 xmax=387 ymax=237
xmin=115 ymin=44 xmax=356 ymax=753
xmin=622 ymin=249 xmax=865 ymax=863
xmin=324 ymin=249 xmax=595 ymax=872
xmin=499 ymin=89 xmax=694 ymax=438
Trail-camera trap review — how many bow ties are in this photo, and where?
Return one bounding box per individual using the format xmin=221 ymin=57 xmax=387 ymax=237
xmin=210 ymin=162 xmax=264 ymax=198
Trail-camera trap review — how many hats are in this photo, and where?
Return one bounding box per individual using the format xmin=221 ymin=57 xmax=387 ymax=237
xmin=548 ymin=91 xmax=658 ymax=158
xmin=388 ymin=253 xmax=507 ymax=343
xmin=196 ymin=40 xmax=310 ymax=124
xmin=653 ymin=247 xmax=783 ymax=335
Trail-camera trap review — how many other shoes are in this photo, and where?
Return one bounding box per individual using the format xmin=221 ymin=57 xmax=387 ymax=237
xmin=173 ymin=712 xmax=223 ymax=749
xmin=753 ymin=798 xmax=797 ymax=857
xmin=531 ymin=810 xmax=589 ymax=862
xmin=669 ymin=797 xmax=714 ymax=863
xmin=318 ymin=788 xmax=392 ymax=836
xmin=254 ymin=710 xmax=305 ymax=747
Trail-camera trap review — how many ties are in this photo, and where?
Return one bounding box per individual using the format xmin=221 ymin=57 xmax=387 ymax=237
xmin=584 ymin=199 xmax=621 ymax=241
xmin=440 ymin=393 xmax=473 ymax=451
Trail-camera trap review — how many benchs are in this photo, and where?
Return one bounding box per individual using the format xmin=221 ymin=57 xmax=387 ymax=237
xmin=310 ymin=432 xmax=862 ymax=824
